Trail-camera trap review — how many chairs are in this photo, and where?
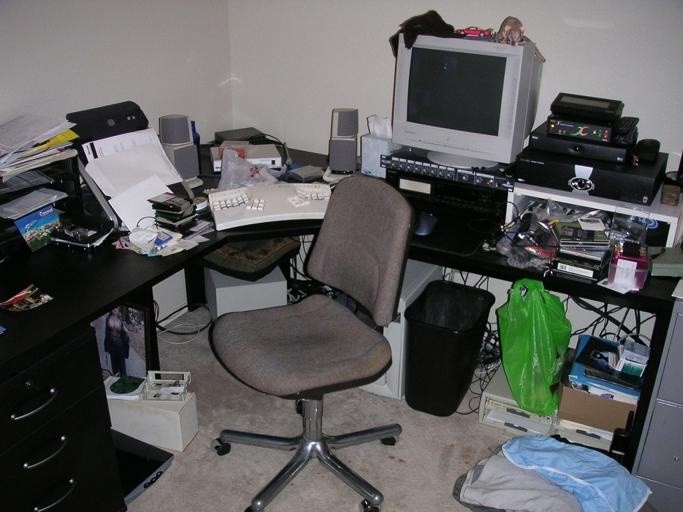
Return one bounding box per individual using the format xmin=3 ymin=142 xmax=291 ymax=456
xmin=210 ymin=173 xmax=405 ymax=510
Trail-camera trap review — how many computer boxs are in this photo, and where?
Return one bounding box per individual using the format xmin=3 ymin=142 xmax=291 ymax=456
xmin=358 ymin=258 xmax=442 ymax=401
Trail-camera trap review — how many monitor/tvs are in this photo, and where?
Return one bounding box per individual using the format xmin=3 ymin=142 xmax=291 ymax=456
xmin=391 ymin=33 xmax=542 ymax=169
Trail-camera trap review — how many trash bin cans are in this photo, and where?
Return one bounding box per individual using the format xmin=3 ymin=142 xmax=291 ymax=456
xmin=404 ymin=280 xmax=495 ymax=417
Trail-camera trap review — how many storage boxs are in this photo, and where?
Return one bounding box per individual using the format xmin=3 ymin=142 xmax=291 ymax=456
xmin=559 ymin=346 xmax=637 ymax=432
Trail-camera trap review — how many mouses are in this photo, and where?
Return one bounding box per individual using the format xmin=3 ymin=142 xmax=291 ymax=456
xmin=413 ymin=208 xmax=439 ymax=236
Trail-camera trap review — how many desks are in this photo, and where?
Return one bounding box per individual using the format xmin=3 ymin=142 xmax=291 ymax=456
xmin=0 ymin=148 xmax=338 ymax=470
xmin=384 ymin=242 xmax=677 ymax=475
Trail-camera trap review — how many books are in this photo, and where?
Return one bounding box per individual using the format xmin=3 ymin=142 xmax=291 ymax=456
xmin=0 ymin=111 xmax=79 ymax=219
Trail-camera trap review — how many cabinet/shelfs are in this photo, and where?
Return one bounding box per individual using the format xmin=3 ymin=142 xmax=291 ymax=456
xmin=0 ymin=327 xmax=124 ymax=512
xmin=632 ymin=313 xmax=681 ymax=512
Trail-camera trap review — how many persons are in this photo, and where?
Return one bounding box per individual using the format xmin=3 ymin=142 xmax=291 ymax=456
xmin=104 ymin=307 xmax=131 ymax=376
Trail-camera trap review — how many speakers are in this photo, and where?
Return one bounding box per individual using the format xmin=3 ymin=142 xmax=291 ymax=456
xmin=329 ymin=109 xmax=358 ymax=172
xmin=160 ymin=115 xmax=200 ymax=180
